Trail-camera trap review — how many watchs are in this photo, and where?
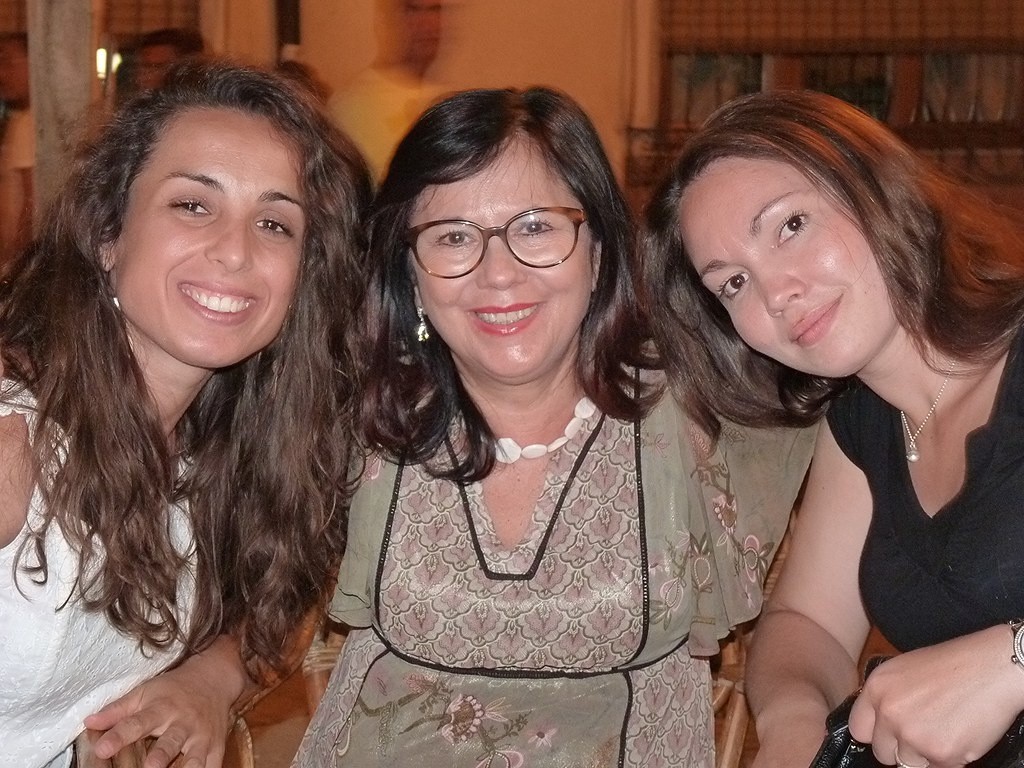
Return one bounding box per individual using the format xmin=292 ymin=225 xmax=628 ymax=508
xmin=1007 ymin=613 xmax=1024 ymax=675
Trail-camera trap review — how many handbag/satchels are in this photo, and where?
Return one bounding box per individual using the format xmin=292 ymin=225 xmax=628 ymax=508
xmin=811 ymin=653 xmax=1023 ymax=768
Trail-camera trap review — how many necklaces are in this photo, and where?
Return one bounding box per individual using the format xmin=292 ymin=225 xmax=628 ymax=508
xmin=901 ymin=358 xmax=962 ymax=463
xmin=451 ymin=398 xmax=596 ymax=462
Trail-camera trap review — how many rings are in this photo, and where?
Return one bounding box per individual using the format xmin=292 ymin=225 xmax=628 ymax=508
xmin=894 ymin=745 xmax=930 ymax=768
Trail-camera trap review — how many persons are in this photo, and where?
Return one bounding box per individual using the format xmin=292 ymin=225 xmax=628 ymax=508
xmin=2 ymin=58 xmax=377 ymax=768
xmin=298 ymin=87 xmax=820 ymax=767
xmin=124 ymin=27 xmax=205 ymax=93
xmin=637 ymin=90 xmax=1024 ymax=767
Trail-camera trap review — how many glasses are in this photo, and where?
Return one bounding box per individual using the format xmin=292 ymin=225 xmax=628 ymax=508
xmin=404 ymin=206 xmax=587 ymax=279
